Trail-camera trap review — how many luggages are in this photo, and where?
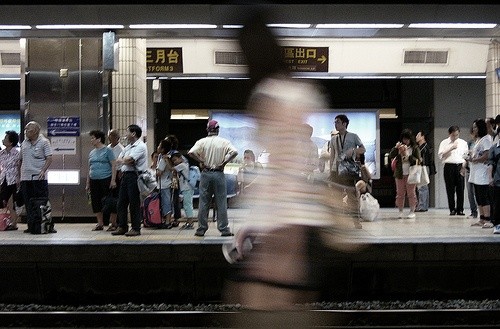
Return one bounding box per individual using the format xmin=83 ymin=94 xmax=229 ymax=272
xmin=143 ymin=192 xmax=162 ymax=227
xmin=26 ymin=174 xmax=54 ymax=234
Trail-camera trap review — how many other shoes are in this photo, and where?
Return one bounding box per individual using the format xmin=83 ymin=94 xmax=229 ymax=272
xmin=414 ymin=208 xmax=428 ymax=212
xmin=111 ymin=227 xmax=128 ymax=235
xmin=353 ymin=218 xmax=362 ymax=229
xmin=407 ymin=213 xmax=415 ymax=219
xmin=106 ymin=225 xmax=116 ymax=231
xmin=24 ymin=228 xmax=30 ymax=233
xmin=468 ymin=214 xmax=477 ymax=219
xmin=221 ymin=232 xmax=234 ymax=236
xmin=471 ymin=220 xmax=494 ymax=228
xmin=450 ymin=210 xmax=465 ymax=215
xmin=158 ymin=221 xmax=173 ymax=229
xmin=5 ymin=225 xmax=18 ymax=230
xmin=50 ymin=229 xmax=57 ymax=233
xmin=195 ymin=232 xmax=204 ymax=236
xmin=125 ymin=228 xmax=141 ymax=236
xmin=398 ymin=213 xmax=404 ymax=218
xmin=179 ymin=220 xmax=194 ymax=230
xmin=92 ymin=224 xmax=104 ymax=231
xmin=173 ymin=220 xmax=180 ymax=227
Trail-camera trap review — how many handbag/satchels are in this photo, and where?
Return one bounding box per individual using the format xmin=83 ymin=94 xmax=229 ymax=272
xmin=360 ymin=193 xmax=379 ymax=222
xmin=418 ymin=165 xmax=430 ymax=189
xmin=407 ymin=160 xmax=421 ymax=184
xmin=0 ymin=206 xmax=11 ymax=230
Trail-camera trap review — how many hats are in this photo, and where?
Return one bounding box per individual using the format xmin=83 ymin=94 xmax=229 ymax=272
xmin=206 ymin=120 xmax=219 ymax=131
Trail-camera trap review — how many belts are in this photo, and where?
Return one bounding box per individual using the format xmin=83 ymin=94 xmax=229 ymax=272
xmin=203 ymin=167 xmax=223 ymax=172
xmin=445 ymin=164 xmax=462 ymax=167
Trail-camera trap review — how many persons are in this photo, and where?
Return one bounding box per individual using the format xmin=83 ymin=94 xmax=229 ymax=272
xmin=389 ymin=128 xmax=422 ymax=219
xmin=228 ymin=20 xmax=325 ymax=312
xmin=140 ymin=136 xmax=195 ymax=229
xmin=103 ymin=128 xmax=125 ymax=227
xmin=438 ymin=126 xmax=469 ymax=216
xmin=355 ymin=153 xmax=372 ymax=199
xmin=188 ymin=119 xmax=239 ymax=237
xmin=300 ymin=122 xmax=320 ymax=185
xmin=86 ymin=130 xmax=117 ymax=231
xmin=16 ymin=121 xmax=58 ymax=233
xmin=414 ymin=131 xmax=436 ymax=212
xmin=326 ymin=114 xmax=366 ymax=230
xmin=460 ymin=118 xmax=493 ymax=228
xmin=487 ymin=126 xmax=500 ymax=235
xmin=111 ymin=125 xmax=148 ymax=236
xmin=237 ymin=149 xmax=265 ymax=203
xmin=485 ymin=114 xmax=500 ymax=140
xmin=0 ymin=131 xmax=21 ymax=230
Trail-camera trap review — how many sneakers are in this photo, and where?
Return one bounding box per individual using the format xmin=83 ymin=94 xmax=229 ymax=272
xmin=493 ymin=224 xmax=500 ymax=234
